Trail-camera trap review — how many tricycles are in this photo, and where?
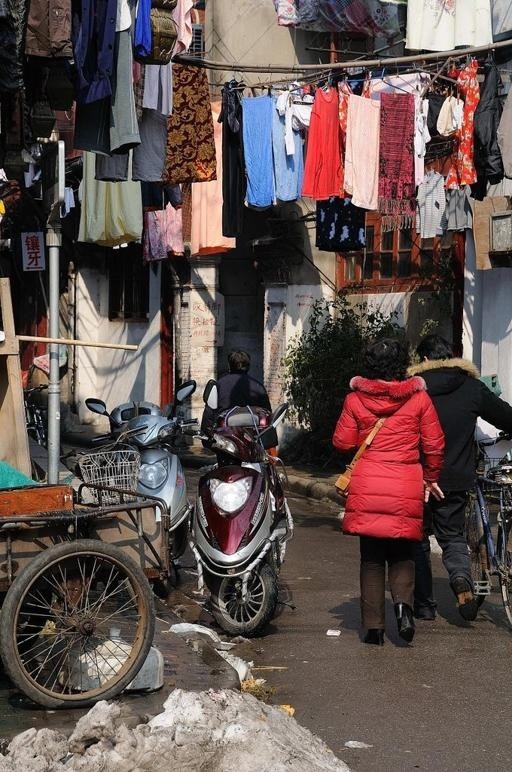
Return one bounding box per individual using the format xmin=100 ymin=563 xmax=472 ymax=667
xmin=1 ymin=484 xmax=170 ymax=710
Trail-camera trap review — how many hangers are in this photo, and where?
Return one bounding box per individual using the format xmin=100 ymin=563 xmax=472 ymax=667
xmin=205 ymin=57 xmax=499 ymax=98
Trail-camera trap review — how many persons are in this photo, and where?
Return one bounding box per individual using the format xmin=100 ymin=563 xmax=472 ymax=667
xmin=406 ymin=336 xmax=512 ymax=621
xmin=200 ymin=351 xmax=278 ymax=464
xmin=333 ymin=333 xmax=447 ymax=647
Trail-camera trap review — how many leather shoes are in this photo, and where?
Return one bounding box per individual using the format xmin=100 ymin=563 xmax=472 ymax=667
xmin=450 ymin=576 xmax=478 ymax=621
xmin=413 ymin=600 xmax=436 ymax=620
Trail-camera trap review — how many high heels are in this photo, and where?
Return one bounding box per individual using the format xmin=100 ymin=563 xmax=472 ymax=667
xmin=364 ymin=629 xmax=385 ymax=646
xmin=394 ymin=602 xmax=416 ymax=642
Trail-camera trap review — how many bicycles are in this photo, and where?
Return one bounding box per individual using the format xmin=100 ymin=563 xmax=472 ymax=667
xmin=465 ymin=430 xmax=512 ymax=628
xmin=23 ymin=384 xmax=49 ymax=446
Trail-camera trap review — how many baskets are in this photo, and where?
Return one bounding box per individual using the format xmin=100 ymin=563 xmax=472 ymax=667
xmin=477 ymin=458 xmax=512 ymax=506
xmin=78 ymin=450 xmax=143 ymax=506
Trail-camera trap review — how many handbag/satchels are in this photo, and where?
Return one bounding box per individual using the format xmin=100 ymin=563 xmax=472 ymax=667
xmin=335 ymin=468 xmax=351 ymax=498
